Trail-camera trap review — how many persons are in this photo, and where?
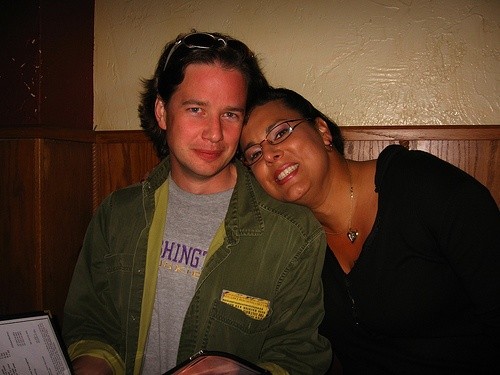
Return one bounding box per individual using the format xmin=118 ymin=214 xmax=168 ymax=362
xmin=62 ymin=32 xmax=327 ymax=375
xmin=240 ymin=88 xmax=500 ymax=375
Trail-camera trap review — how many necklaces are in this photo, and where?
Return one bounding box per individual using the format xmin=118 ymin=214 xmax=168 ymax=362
xmin=322 ymin=158 xmax=359 ymax=243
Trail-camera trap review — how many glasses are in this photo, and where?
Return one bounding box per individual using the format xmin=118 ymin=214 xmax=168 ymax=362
xmin=163 ymin=32 xmax=254 ymax=71
xmin=240 ymin=118 xmax=312 ymax=167
